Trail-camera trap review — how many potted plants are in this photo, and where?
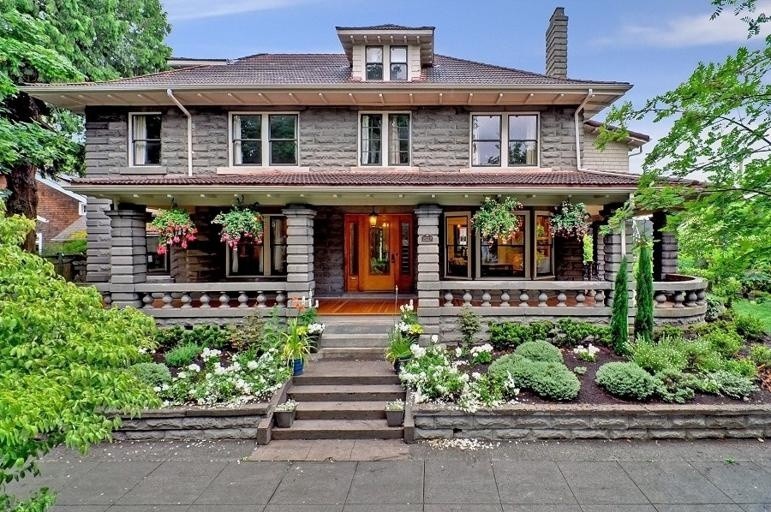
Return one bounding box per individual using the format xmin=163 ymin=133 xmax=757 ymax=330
xmin=384 ymin=308 xmax=425 ymax=374
xmin=278 ymin=308 xmax=326 ymax=376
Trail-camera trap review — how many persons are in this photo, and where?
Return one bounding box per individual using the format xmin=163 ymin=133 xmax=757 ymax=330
xmin=488 ymin=236 xmax=498 ymax=259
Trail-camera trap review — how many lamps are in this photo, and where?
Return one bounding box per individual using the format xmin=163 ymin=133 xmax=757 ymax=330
xmin=369 ymin=206 xmax=378 ymax=228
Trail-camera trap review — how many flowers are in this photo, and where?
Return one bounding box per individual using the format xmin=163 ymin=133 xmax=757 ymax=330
xmin=148 ymin=209 xmax=198 ymax=256
xmin=211 ymin=206 xmax=265 ymax=249
xmin=471 ymin=194 xmax=525 ymax=249
xmin=548 ymin=201 xmax=593 ymax=242
